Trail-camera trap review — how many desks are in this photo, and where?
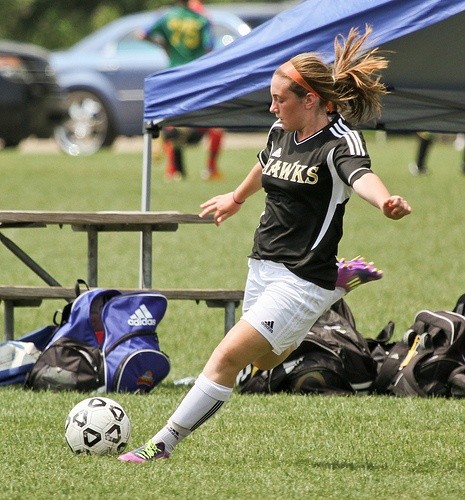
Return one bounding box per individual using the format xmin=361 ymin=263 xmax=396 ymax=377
xmin=0 ymin=211 xmax=213 ymax=288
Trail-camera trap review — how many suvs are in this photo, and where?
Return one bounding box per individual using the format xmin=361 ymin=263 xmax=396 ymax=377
xmin=1 ymin=34 xmax=64 ymax=150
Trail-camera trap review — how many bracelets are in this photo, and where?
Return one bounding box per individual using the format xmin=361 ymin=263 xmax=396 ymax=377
xmin=233 ymin=193 xmax=245 ymax=204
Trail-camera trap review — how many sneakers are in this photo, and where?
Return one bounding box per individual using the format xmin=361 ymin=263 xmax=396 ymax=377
xmin=117 ymin=440 xmax=170 ymax=462
xmin=336 ymin=256 xmax=384 ymax=292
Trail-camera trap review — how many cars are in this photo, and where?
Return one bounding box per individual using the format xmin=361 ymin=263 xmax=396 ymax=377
xmin=49 ymin=2 xmax=268 ymax=160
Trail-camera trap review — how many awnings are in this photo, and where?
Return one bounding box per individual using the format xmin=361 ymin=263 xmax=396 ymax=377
xmin=139 ymin=0 xmax=464 ymax=290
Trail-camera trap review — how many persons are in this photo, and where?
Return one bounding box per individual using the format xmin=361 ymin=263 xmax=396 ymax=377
xmin=142 ymin=0 xmax=224 ymax=184
xmin=413 ymin=132 xmax=465 ymax=175
xmin=118 ymin=21 xmax=411 ymax=464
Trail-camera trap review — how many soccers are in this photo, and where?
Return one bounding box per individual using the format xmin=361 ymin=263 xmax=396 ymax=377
xmin=66 ymin=396 xmax=131 ymax=459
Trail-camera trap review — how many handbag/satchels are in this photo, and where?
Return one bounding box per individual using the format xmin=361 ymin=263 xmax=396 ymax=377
xmin=24 ymin=337 xmax=104 ymax=392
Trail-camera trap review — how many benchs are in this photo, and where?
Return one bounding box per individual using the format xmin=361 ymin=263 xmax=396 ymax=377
xmin=0 ymin=287 xmax=245 ymax=341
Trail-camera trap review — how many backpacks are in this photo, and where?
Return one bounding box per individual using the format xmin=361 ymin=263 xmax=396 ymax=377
xmin=42 ymin=278 xmax=171 ymax=392
xmin=236 ymin=295 xmax=464 ymax=397
